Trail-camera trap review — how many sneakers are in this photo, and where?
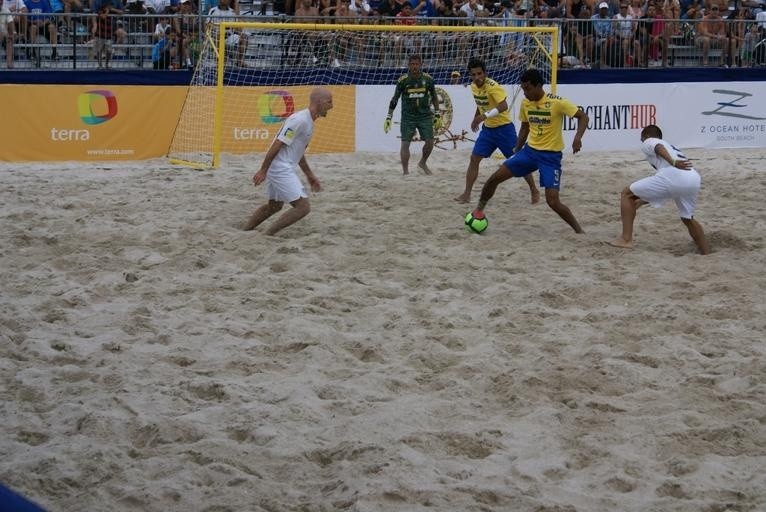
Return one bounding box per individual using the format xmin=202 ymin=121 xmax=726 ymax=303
xmin=329 ymin=59 xmax=340 ymax=68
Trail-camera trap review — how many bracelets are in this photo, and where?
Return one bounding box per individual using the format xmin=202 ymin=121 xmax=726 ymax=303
xmin=483 ymin=108 xmax=500 ymax=119
xmin=673 ymin=159 xmax=677 ymax=167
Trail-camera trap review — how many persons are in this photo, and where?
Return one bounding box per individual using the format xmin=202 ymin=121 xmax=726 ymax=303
xmin=476 ymin=69 xmax=590 ymax=236
xmin=243 ymin=87 xmax=336 ymax=239
xmin=1 ymin=0 xmax=766 ymax=83
xmin=452 ymin=59 xmax=541 ymax=205
xmin=384 ymin=56 xmax=443 ymax=176
xmin=606 ymin=124 xmax=710 ymax=255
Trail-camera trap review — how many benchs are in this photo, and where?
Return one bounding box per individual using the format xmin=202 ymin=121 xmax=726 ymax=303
xmin=12 ymin=27 xmax=154 ymax=69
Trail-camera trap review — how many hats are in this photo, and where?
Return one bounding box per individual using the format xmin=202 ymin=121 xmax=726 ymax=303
xmin=599 ymin=2 xmax=609 ymax=9
xmin=711 ymin=4 xmax=719 ymax=9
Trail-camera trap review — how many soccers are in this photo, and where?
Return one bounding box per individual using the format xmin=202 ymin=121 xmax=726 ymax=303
xmin=465 ymin=212 xmax=489 ymax=235
